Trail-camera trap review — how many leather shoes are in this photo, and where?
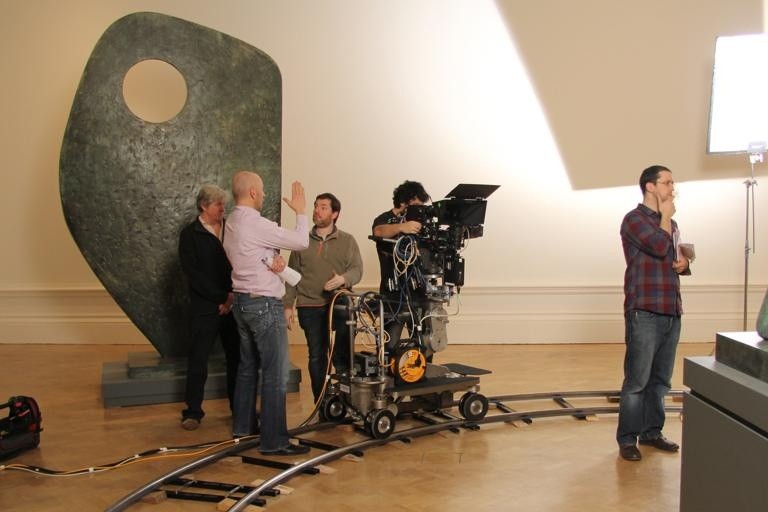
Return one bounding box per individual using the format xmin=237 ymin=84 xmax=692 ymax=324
xmin=619 ymin=444 xmax=641 ymax=461
xmin=639 ymin=432 xmax=679 ymax=452
xmin=263 ymin=439 xmax=310 ymax=455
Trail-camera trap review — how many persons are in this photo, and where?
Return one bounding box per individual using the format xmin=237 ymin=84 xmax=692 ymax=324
xmin=179 ymin=183 xmax=234 ymax=431
xmin=617 ymin=165 xmax=695 ymax=461
xmin=372 ymin=181 xmax=432 ymax=366
xmin=222 ymin=170 xmax=310 ymax=456
xmin=283 ymin=193 xmax=364 ymax=424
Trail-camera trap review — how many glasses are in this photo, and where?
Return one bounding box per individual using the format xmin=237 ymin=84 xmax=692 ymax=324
xmin=659 ymin=180 xmax=673 ymax=185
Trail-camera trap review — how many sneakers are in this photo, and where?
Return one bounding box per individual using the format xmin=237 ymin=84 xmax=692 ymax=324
xmin=232 ymin=408 xmax=260 ymax=417
xmin=182 ymin=418 xmax=197 ymax=429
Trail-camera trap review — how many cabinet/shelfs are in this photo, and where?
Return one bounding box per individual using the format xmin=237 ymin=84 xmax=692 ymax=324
xmin=675 ymin=388 xmax=767 ymax=512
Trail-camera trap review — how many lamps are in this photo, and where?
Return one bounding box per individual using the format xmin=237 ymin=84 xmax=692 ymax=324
xmin=705 ymin=32 xmax=768 ymax=331
